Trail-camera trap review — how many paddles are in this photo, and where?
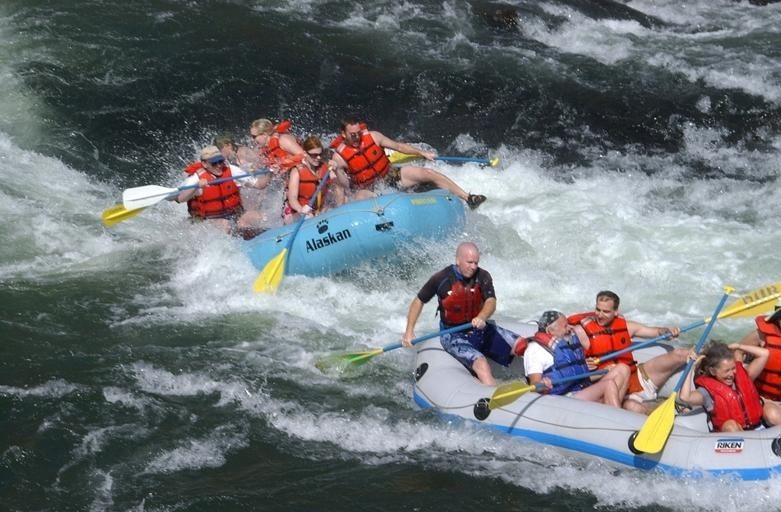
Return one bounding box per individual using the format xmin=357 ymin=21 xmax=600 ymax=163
xmin=122 ymin=168 xmax=273 ymax=212
xmin=488 ymin=369 xmax=608 ymax=412
xmin=633 ymin=285 xmax=735 ymax=454
xmin=314 ymin=322 xmax=473 ymax=377
xmin=102 ymin=205 xmax=145 ymax=226
xmin=251 ymin=166 xmax=333 ymax=296
xmin=594 ymin=280 xmax=781 ymax=366
xmin=388 ymin=151 xmax=499 ymax=168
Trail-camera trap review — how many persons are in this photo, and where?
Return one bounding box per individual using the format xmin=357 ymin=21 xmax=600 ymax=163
xmin=524 ymin=311 xmax=631 ymax=409
xmin=402 ymin=241 xmax=524 ymax=387
xmin=251 ymin=119 xmax=305 ymax=183
xmin=213 ymin=135 xmax=259 ymax=169
xmin=733 ymin=307 xmax=781 ymax=426
xmin=573 ymin=290 xmax=706 ymax=414
xmin=679 ymin=342 xmax=769 ymax=432
xmin=331 ymin=116 xmax=487 ymax=211
xmin=280 ymin=136 xmax=349 ymax=224
xmin=174 ymin=146 xmax=279 ymax=236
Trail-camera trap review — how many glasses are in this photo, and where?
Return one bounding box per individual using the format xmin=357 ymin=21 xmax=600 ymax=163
xmin=306 ymin=151 xmax=324 ymax=160
xmin=348 ymin=132 xmax=364 ymax=138
xmin=250 ymin=134 xmax=265 ymax=139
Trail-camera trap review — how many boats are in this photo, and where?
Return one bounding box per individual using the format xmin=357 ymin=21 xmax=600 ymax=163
xmin=412 ymin=324 xmax=781 ymax=481
xmin=240 ymin=190 xmax=466 ymax=277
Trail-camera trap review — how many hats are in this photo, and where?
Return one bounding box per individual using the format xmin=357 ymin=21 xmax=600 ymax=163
xmin=199 ymin=146 xmax=227 ymax=165
